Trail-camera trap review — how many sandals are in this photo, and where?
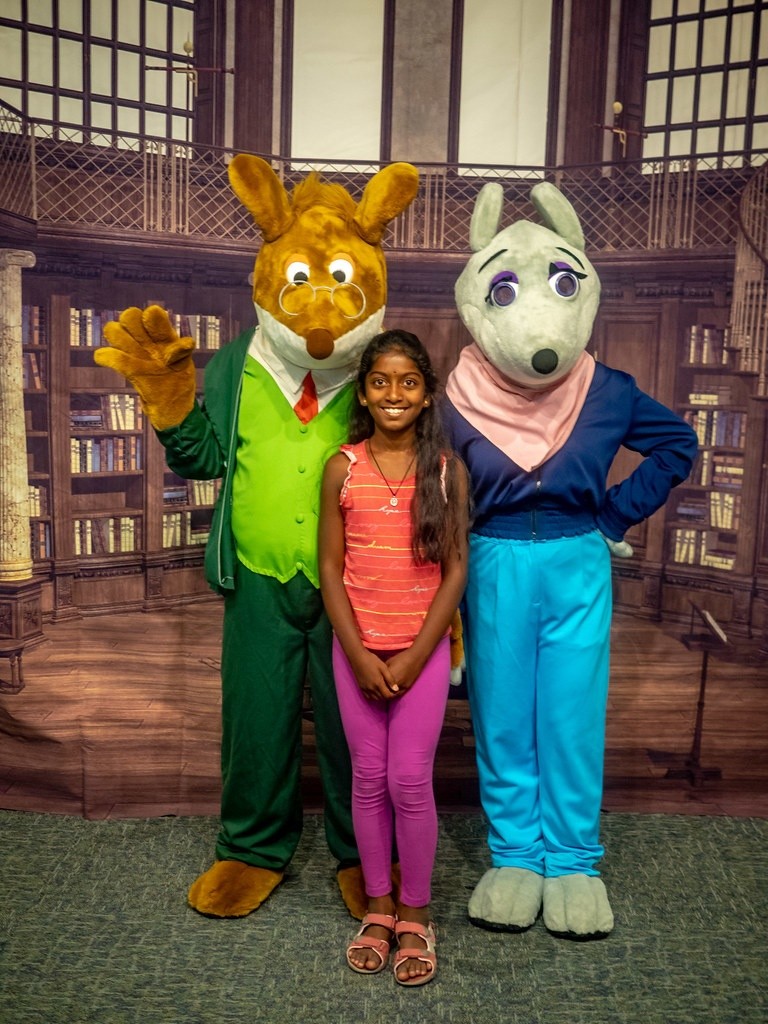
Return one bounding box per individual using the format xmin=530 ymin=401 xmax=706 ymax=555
xmin=346 ymin=913 xmax=399 ymax=974
xmin=393 ymin=915 xmax=437 ymax=987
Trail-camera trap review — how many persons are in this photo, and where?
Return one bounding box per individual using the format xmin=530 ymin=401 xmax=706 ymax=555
xmin=317 ymin=327 xmax=468 ymax=986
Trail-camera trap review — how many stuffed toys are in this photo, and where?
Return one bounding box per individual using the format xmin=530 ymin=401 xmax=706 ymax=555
xmin=94 ymin=154 xmax=464 ymax=928
xmin=437 ymin=179 xmax=700 ymax=934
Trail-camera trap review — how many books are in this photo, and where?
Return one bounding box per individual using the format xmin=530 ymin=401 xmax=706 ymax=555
xmin=667 ymin=320 xmax=748 ymax=574
xmin=20 ymin=297 xmax=222 ymax=562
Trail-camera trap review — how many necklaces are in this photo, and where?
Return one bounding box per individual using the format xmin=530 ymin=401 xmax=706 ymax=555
xmin=368 ymin=438 xmax=419 ymax=506
